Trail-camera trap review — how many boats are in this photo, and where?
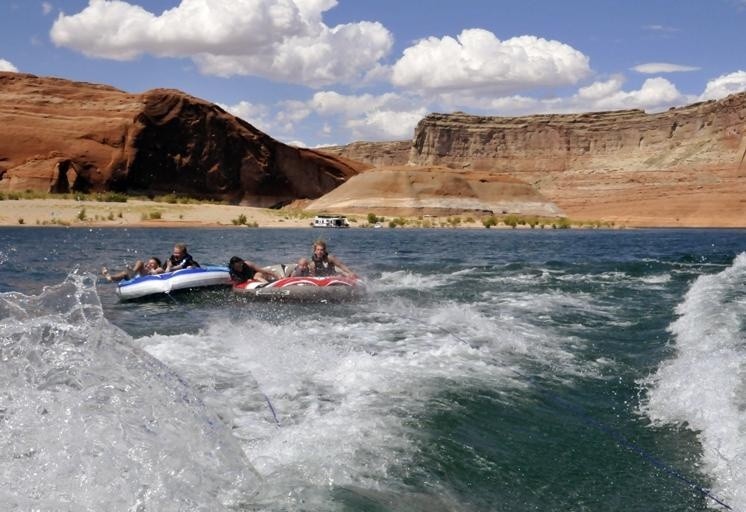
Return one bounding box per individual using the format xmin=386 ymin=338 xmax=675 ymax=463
xmin=114 ymin=260 xmax=235 ymax=302
xmin=231 ymin=258 xmax=369 ymax=302
xmin=312 ymin=215 xmax=352 ymax=228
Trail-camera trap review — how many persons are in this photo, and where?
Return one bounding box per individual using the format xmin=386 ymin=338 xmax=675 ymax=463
xmin=228 ymin=256 xmax=284 ymax=287
xmin=289 ymin=257 xmax=310 ymax=278
xmin=308 ymin=239 xmax=359 ymax=279
xmin=102 ymin=257 xmax=166 ymax=281
xmin=162 ymin=244 xmax=202 ymax=273
